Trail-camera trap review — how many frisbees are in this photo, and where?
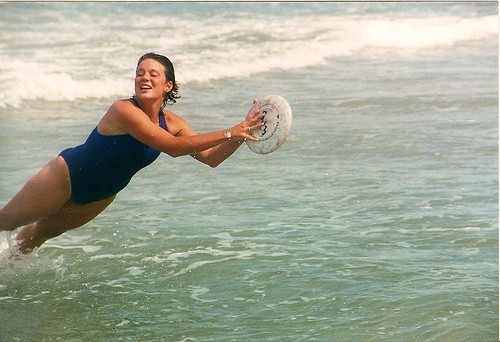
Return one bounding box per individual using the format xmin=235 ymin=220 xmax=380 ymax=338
xmin=246 ymin=94 xmax=292 ymax=155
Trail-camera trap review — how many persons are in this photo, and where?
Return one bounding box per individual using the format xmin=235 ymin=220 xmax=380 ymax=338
xmin=0 ymin=53 xmax=266 ymax=256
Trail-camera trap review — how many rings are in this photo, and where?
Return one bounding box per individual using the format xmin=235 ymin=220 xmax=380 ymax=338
xmin=242 ymin=135 xmax=249 ymax=141
xmin=248 ymin=126 xmax=252 ymax=132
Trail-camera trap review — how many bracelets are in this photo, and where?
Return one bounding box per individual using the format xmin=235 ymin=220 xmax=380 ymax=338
xmin=225 ymin=128 xmax=232 ymax=141
